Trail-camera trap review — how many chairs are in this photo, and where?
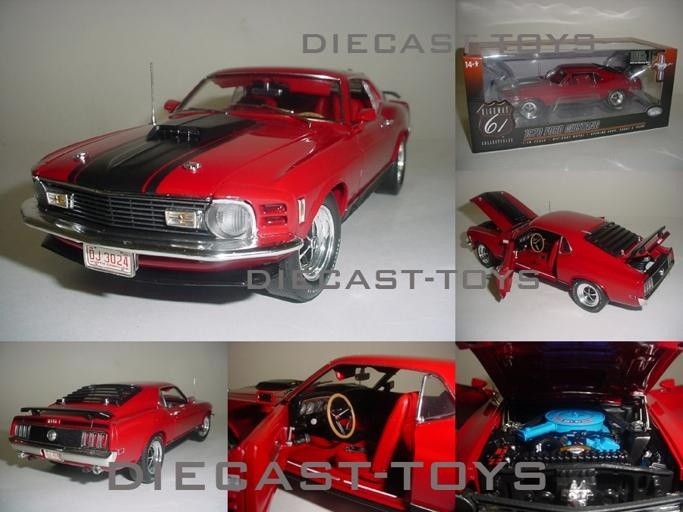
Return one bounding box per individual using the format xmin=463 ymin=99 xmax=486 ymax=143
xmin=236 ymin=76 xmax=368 ymax=127
xmin=347 ymin=391 xmax=419 ymax=482
xmin=328 ymin=394 xmax=412 ymax=492
